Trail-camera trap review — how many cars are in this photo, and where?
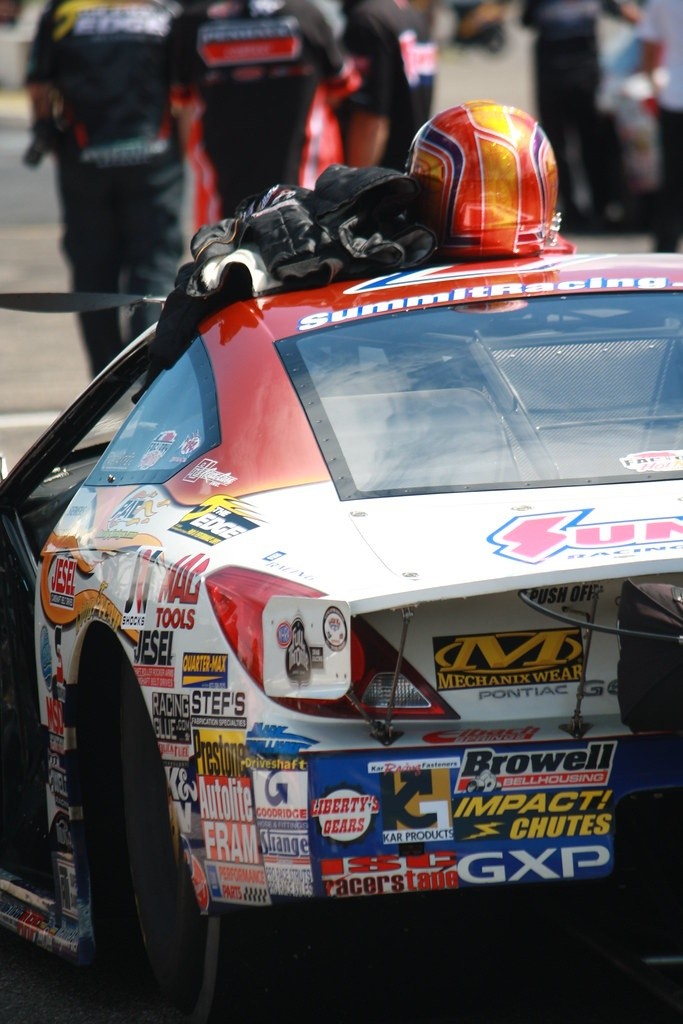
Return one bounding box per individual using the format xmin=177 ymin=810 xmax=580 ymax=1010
xmin=0 ymin=253 xmax=682 ymax=1023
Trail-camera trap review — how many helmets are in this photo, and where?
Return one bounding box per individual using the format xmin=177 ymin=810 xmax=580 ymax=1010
xmin=404 ymin=101 xmax=559 ymax=256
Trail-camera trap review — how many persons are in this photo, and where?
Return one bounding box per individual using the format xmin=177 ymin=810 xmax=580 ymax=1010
xmin=633 ymin=0 xmax=683 ymax=251
xmin=23 ymin=0 xmax=210 ymax=373
xmin=171 ymin=0 xmax=363 ymax=216
xmin=516 ymin=0 xmax=647 ymax=232
xmin=336 ymin=0 xmax=436 ymax=174
